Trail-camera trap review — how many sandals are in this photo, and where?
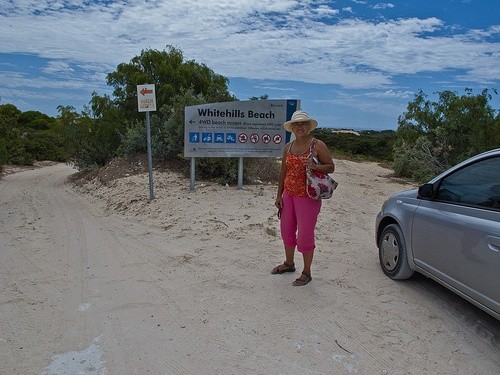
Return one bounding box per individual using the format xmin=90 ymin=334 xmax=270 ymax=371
xmin=271 ymin=261 xmax=295 ymax=273
xmin=293 ymin=271 xmax=312 ymax=286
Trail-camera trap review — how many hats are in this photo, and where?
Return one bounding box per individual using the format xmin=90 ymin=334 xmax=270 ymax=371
xmin=284 ymin=110 xmax=318 ymax=132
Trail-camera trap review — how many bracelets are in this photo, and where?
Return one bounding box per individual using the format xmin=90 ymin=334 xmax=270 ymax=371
xmin=314 ymin=164 xmax=318 ymax=170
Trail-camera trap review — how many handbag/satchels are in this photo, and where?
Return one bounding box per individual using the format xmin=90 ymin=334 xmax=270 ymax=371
xmin=306 ymin=138 xmax=338 ymax=200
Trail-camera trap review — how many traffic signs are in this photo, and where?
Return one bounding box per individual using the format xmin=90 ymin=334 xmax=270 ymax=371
xmin=136 ymin=84 xmax=158 ymax=112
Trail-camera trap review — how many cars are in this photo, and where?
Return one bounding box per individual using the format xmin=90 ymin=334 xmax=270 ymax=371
xmin=374 ymin=147 xmax=500 ymax=320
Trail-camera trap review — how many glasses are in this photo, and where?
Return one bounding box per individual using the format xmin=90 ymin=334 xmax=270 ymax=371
xmin=277 ymin=206 xmax=282 ymax=219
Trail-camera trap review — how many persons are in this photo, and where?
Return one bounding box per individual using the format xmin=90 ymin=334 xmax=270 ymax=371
xmin=272 ymin=110 xmax=335 ymax=286
